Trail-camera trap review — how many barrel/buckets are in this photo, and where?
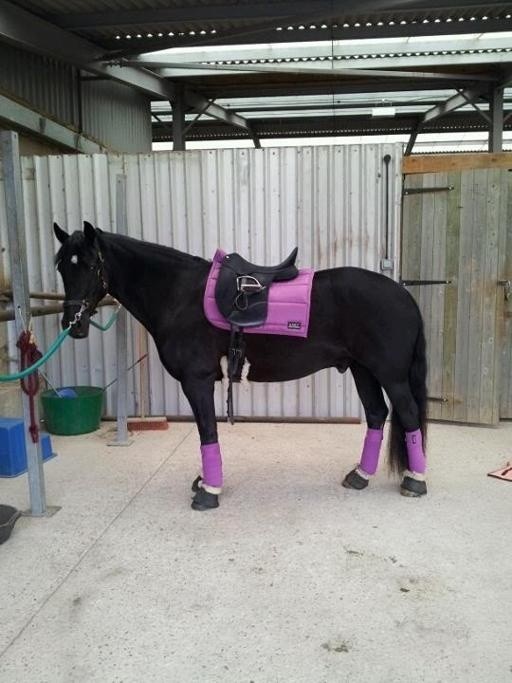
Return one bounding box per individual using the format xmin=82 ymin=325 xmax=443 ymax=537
xmin=40 ymin=385 xmax=105 ymax=436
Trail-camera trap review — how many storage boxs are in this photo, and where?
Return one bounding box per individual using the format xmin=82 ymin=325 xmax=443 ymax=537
xmin=0 ymin=417 xmax=52 ymax=478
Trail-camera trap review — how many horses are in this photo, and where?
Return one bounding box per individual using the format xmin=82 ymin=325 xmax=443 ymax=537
xmin=52 ymin=220 xmax=429 ymax=511
